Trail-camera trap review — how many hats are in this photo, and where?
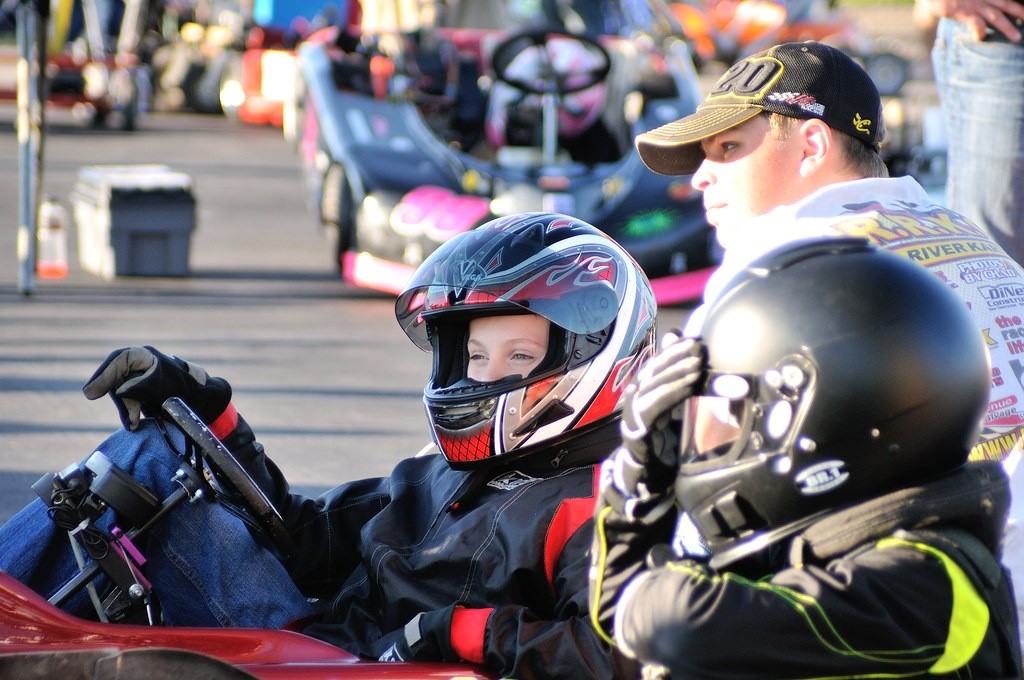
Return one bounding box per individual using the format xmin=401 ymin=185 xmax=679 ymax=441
xmin=636 ymin=41 xmax=885 ymax=175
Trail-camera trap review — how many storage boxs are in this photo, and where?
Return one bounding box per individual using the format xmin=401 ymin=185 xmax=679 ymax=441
xmin=69 ymin=163 xmax=199 ymax=282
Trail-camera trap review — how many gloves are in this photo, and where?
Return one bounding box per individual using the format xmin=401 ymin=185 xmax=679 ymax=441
xmin=611 ymin=326 xmax=707 ymax=504
xmin=365 ymin=601 xmax=468 ymax=664
xmin=82 ymin=345 xmax=233 ymax=432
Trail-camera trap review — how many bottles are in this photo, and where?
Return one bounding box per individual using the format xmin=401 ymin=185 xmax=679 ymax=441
xmin=35 ymin=194 xmax=70 ymax=280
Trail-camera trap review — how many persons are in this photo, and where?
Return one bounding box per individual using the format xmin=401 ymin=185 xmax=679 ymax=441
xmin=585 ymin=0 xmax=1024 ymax=680
xmin=0 ymin=213 xmax=656 ymax=680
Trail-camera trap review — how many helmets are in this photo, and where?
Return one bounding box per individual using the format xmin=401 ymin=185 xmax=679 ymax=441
xmin=688 ymin=236 xmax=991 ymax=586
xmin=396 ymin=211 xmax=661 ymax=472
xmin=487 ymin=36 xmax=610 ymax=149
